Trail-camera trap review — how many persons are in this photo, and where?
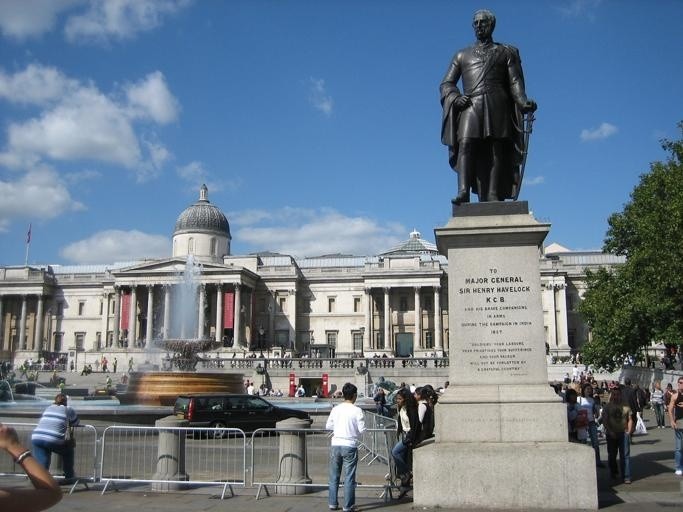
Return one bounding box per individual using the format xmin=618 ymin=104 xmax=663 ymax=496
xmin=202 ymin=353 xmax=449 ymax=512
xmin=550 ymin=342 xmax=683 ymax=484
xmin=441 ymin=9 xmax=537 ymax=206
xmin=0 ymin=352 xmax=183 ymax=511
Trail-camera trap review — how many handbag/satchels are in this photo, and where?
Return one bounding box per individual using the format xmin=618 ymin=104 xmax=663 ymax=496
xmin=64 ymin=405 xmax=76 ymax=448
xmin=675 ymin=420 xmax=683 ymax=430
xmin=631 ymin=411 xmax=648 ymax=435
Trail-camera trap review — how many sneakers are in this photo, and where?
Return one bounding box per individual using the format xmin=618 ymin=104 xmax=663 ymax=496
xmin=397 ymin=471 xmax=413 ymax=501
xmin=344 ymin=505 xmax=360 ymax=512
xmin=327 ymin=502 xmax=338 ymax=509
xmin=595 ymin=462 xmax=631 ymax=484
xmin=674 ymin=470 xmax=682 ymax=475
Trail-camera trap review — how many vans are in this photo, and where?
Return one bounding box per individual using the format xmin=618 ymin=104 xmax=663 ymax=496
xmin=174 ymin=390 xmax=313 ymax=440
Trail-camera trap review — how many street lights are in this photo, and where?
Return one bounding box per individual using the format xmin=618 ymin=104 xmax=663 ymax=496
xmin=309 ymin=335 xmax=314 ymax=345
xmin=258 ymin=320 xmax=265 ymax=359
xmin=356 ymin=325 xmax=366 ymax=358
